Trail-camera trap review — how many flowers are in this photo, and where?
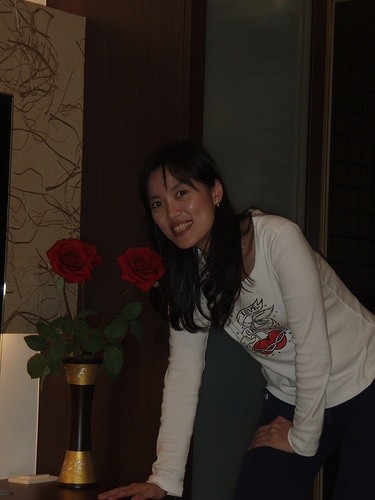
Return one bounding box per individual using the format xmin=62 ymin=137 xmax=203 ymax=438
xmin=23 ymin=237 xmax=166 ymax=392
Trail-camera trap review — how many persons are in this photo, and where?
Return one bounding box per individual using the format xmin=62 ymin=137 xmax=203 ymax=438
xmin=97 ymin=141 xmax=375 ymax=500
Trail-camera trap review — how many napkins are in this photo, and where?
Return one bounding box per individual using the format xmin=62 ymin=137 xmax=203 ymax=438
xmin=8 ymin=474 xmax=59 ymax=485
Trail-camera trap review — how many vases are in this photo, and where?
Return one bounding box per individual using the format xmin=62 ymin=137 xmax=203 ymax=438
xmin=57 ymin=357 xmax=104 ymax=491
